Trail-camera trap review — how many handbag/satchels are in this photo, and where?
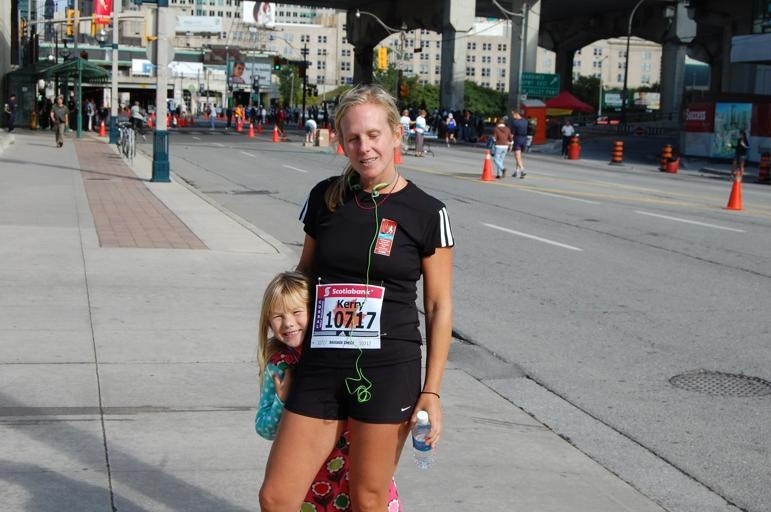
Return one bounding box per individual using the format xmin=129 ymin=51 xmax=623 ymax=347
xmin=487 ymin=136 xmax=495 ymax=155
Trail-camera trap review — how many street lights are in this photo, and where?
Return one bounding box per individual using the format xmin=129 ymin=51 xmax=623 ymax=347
xmin=357 ymin=11 xmax=408 ymax=106
xmin=597 ymin=55 xmax=607 ymax=115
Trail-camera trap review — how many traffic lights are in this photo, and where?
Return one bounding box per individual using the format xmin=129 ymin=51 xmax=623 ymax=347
xmin=274 ymin=56 xmax=279 ymax=70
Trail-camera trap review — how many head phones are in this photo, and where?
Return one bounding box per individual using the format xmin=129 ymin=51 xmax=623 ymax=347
xmin=347 ymin=166 xmax=397 ymax=198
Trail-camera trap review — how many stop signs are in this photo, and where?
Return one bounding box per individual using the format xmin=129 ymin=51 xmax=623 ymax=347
xmin=633 ymin=126 xmax=644 ymax=136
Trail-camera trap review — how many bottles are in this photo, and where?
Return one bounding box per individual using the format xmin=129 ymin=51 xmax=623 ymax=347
xmin=410 ymin=410 xmax=434 ymax=470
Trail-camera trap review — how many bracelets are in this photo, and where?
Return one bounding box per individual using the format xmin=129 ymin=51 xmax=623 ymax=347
xmin=419 ymin=391 xmax=440 ymax=398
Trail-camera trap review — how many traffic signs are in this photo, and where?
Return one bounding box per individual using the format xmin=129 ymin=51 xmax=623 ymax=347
xmin=517 ymin=72 xmax=560 ymax=98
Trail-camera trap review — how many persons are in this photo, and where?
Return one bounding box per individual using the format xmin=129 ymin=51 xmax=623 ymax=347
xmin=257 ymin=86 xmax=456 ymax=511
xmin=118 ymin=100 xmax=156 ymax=141
xmin=304 ymin=105 xmax=319 ymax=144
xmin=489 ymin=109 xmax=535 ymax=179
xmin=39 ymin=93 xmax=109 ymax=148
xmin=229 ymin=61 xmax=245 ymax=87
xmin=725 ymin=130 xmax=752 ymax=182
xmin=3 ymin=94 xmax=18 ymax=133
xmin=167 ymin=99 xmax=302 ymax=135
xmin=262 ymin=4 xmax=274 ymax=26
xmin=457 ymin=109 xmax=484 ymax=143
xmin=256 ymin=270 xmax=403 ymax=512
xmin=398 ymin=98 xmax=456 ymax=157
xmin=560 ymin=120 xmax=575 ymax=158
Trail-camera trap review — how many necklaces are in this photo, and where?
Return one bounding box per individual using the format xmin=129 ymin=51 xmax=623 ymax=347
xmin=354 ymin=172 xmax=400 ymax=210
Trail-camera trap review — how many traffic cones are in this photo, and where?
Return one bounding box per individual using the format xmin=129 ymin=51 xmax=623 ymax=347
xmin=480 ymin=150 xmax=493 ymax=181
xmin=205 ymin=114 xmax=235 ymax=122
xmin=273 ymin=126 xmax=278 ymax=141
xmin=722 ymin=168 xmax=744 ymax=210
xmin=148 ymin=112 xmax=188 ymax=127
xmin=237 ymin=119 xmax=262 ymax=137
xmin=99 ymin=120 xmax=105 ymax=136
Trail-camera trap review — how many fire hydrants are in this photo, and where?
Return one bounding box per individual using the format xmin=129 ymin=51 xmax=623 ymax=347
xmin=31 ymin=112 xmax=37 ymax=130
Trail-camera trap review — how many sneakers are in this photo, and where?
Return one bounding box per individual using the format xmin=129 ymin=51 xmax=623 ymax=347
xmin=496 ymin=168 xmax=526 ymax=178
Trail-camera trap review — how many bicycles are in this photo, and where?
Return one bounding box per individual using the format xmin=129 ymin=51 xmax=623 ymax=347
xmin=118 ymin=121 xmax=136 ymax=160
